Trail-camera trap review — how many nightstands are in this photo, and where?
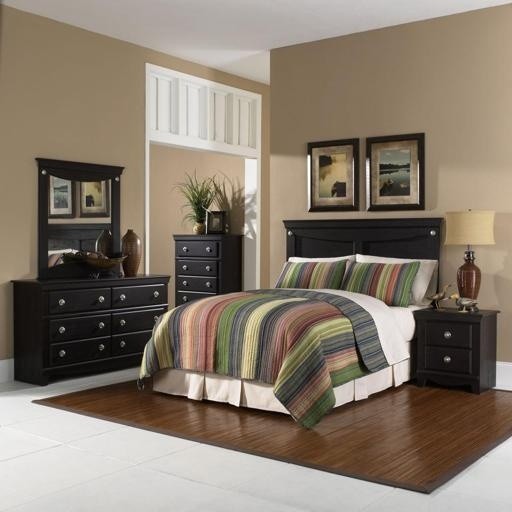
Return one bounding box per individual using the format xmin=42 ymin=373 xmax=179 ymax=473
xmin=411 ymin=306 xmax=501 ymax=395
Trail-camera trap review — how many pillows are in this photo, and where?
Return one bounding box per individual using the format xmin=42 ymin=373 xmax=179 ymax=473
xmin=273 ymin=258 xmax=349 ymax=289
xmin=340 ymin=259 xmax=421 ymax=309
xmin=288 ymin=255 xmax=355 ymax=279
xmin=355 ymin=253 xmax=438 ymax=305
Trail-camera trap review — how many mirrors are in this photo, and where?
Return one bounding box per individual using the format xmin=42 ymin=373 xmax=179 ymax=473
xmin=35 ymin=157 xmax=125 ymax=280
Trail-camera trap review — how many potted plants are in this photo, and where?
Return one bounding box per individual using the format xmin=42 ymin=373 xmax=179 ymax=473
xmin=169 ymin=167 xmax=220 ymax=234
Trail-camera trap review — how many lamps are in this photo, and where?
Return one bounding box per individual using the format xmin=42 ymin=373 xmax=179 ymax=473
xmin=443 ymin=208 xmax=496 ymax=314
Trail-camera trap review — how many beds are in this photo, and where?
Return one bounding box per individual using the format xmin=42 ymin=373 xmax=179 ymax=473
xmin=136 ymin=217 xmax=446 ymax=430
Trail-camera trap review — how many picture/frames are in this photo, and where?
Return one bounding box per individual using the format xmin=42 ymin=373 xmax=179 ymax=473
xmin=207 ymin=211 xmax=226 ymax=234
xmin=50 ymin=175 xmax=75 ymax=219
xmin=306 ymin=138 xmax=360 ymax=213
xmin=80 ymin=180 xmax=110 ymax=218
xmin=365 ymin=133 xmax=426 ymax=211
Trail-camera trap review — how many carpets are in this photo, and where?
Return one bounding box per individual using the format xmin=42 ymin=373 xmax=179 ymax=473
xmin=30 ymin=378 xmax=512 ymax=495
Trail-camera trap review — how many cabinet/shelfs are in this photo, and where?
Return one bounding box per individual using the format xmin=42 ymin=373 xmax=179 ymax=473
xmin=10 ymin=279 xmax=171 ymax=387
xmin=173 ymin=233 xmax=244 ymax=307
